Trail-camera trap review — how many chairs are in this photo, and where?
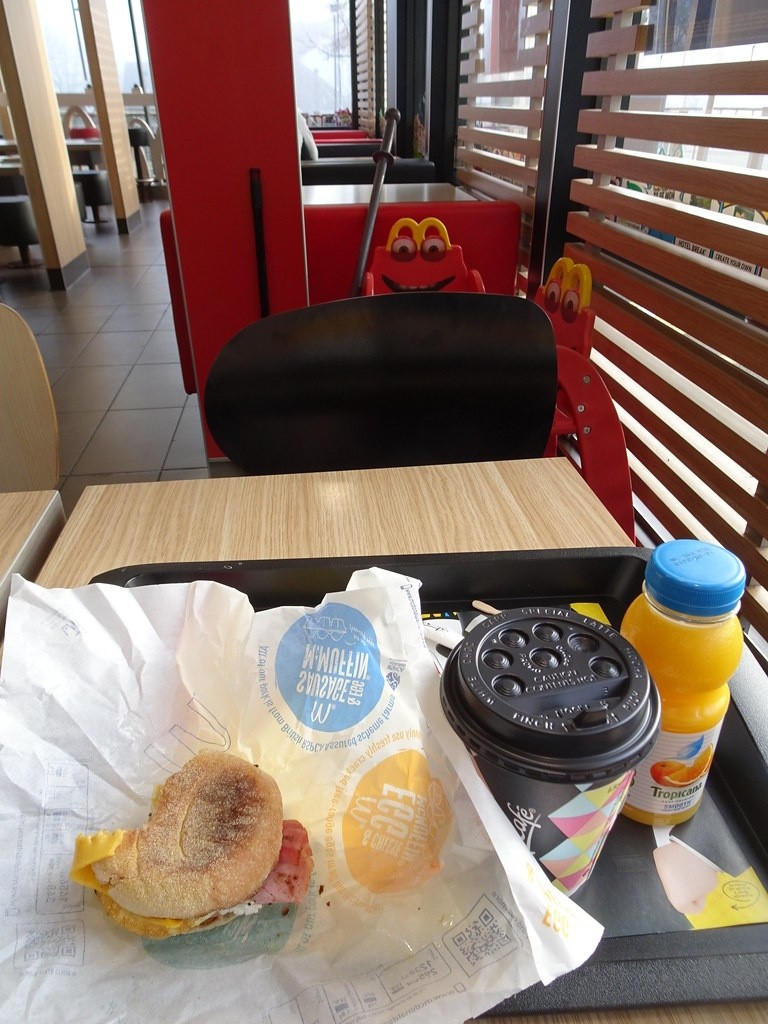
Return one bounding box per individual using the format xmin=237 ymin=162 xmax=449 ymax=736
xmin=0 ymin=303 xmax=60 ymax=493
xmin=0 ymin=127 xmax=146 ymax=270
xmin=204 ymin=292 xmax=560 ymax=476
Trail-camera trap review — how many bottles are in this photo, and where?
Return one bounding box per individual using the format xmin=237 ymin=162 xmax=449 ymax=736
xmin=620 ymin=538 xmax=746 ymax=825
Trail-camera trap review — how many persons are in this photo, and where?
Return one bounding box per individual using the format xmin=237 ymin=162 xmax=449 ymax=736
xmin=296 ymin=111 xmax=320 ymax=185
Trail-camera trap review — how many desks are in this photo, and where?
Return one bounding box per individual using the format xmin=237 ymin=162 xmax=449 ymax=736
xmin=33 ymin=457 xmax=768 ymax=1024
xmin=0 ymin=137 xmax=114 ymax=223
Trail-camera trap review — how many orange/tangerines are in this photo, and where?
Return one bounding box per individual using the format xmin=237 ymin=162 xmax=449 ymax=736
xmin=651 ymin=746 xmax=714 ymax=788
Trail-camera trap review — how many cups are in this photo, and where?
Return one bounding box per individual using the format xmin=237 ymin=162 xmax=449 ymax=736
xmin=438 ymin=609 xmax=662 ymax=903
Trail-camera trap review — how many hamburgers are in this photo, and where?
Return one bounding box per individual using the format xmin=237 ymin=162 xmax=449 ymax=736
xmin=67 ymin=749 xmax=313 ymax=937
